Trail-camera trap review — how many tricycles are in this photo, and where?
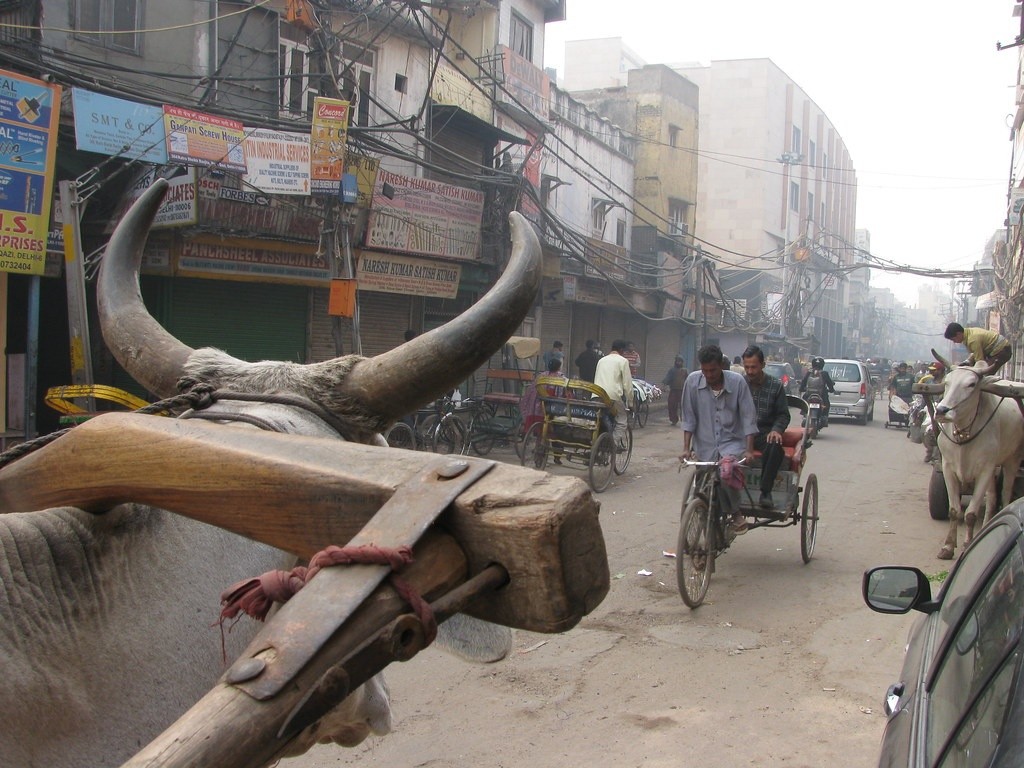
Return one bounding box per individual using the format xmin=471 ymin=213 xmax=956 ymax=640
xmin=518 ymin=374 xmax=635 ymax=494
xmin=672 ymin=392 xmax=821 ymax=610
xmin=429 ymin=335 xmax=541 ymax=457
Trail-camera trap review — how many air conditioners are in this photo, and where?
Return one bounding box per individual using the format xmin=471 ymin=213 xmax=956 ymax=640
xmin=668 ymin=222 xmax=689 ymax=239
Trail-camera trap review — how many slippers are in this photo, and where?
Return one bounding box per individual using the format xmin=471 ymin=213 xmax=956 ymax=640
xmin=731 ymin=512 xmax=748 ymax=535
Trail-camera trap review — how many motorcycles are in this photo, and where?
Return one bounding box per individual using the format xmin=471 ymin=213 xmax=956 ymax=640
xmin=799 ymin=388 xmax=835 ymax=438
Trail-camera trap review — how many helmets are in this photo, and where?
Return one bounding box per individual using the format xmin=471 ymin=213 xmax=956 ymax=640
xmin=812 ymin=358 xmax=825 ymax=369
xmin=899 ymin=362 xmax=907 ymax=368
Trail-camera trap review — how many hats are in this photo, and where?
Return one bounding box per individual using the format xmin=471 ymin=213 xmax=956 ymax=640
xmin=928 ymin=362 xmax=944 ymax=370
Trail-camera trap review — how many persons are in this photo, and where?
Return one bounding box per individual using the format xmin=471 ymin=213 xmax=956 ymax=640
xmin=535 ymin=358 xmax=567 ymax=466
xmin=720 ymin=353 xmax=746 ymax=376
xmin=944 ymin=323 xmax=1013 ymax=376
xmin=800 ymin=357 xmax=834 ymax=428
xmin=542 ymin=341 xmax=565 ymax=372
xmin=592 ymin=340 xmax=634 ymax=450
xmin=738 ymin=345 xmax=791 ymax=508
xmin=663 ymin=355 xmax=689 ymax=425
xmin=621 ymin=342 xmax=641 ymax=378
xmin=405 ymin=331 xmax=417 ymax=340
xmin=678 ymin=344 xmax=759 ymax=536
xmin=575 ymin=340 xmax=601 ymax=400
xmin=842 ymin=355 xmax=947 ymax=428
xmin=594 ymin=342 xmax=605 ymax=357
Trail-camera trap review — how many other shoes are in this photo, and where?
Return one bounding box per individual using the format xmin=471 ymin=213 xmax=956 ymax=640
xmin=823 ymin=416 xmax=828 ymax=427
xmin=801 ymin=417 xmax=807 ymax=428
xmin=759 ymin=492 xmax=774 ymax=509
xmin=671 ymin=416 xmax=679 ymax=425
xmin=553 ymin=455 xmax=563 ymax=465
xmin=616 ymin=445 xmax=628 ymax=454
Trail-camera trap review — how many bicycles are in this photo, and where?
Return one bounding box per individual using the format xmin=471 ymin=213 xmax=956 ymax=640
xmin=383 ymin=398 xmax=454 ymax=455
xmin=626 ymin=398 xmax=650 ymax=429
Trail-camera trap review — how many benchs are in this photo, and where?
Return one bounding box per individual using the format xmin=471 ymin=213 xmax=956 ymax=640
xmin=750 ymin=425 xmax=806 ymax=470
xmin=545 ymin=396 xmax=614 ymax=432
xmin=483 ymin=369 xmax=537 ymax=408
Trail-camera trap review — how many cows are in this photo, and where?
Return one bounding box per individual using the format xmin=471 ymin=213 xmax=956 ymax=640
xmin=930 ymin=349 xmax=1024 ymax=560
xmin=0 ymin=177 xmax=547 ymax=768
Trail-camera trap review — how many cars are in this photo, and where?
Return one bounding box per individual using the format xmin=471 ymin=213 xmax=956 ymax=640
xmin=863 ymin=495 xmax=1024 ymax=768
xmin=760 ymin=361 xmax=802 ymax=397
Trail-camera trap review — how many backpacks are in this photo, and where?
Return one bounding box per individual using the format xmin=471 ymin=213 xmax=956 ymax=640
xmin=805 ymin=370 xmax=825 ymax=395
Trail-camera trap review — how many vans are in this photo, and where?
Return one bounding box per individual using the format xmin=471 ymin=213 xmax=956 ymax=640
xmin=820 ymin=359 xmax=878 ymax=425
